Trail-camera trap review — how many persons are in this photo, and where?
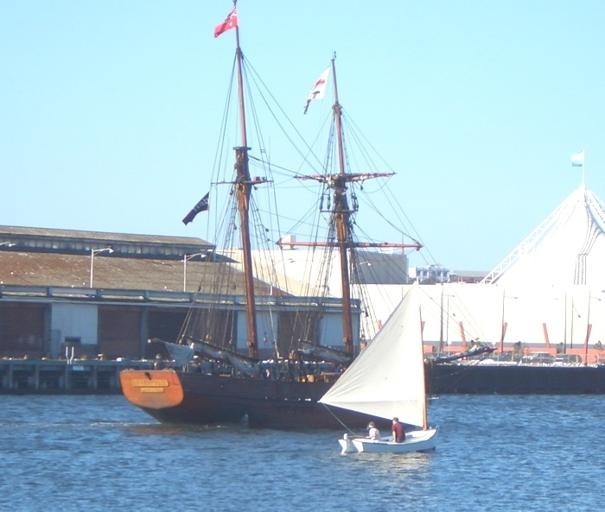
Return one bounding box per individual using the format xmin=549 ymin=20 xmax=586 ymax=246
xmin=152 ymin=353 xmax=166 ymax=370
xmin=392 ymin=418 xmax=405 ymax=443
xmin=366 ymin=422 xmax=381 ymax=441
xmin=187 ymin=355 xmax=200 ymax=375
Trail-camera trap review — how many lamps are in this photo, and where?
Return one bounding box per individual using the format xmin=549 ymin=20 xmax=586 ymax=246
xmin=0 ymin=240 xmax=17 ymax=254
xmin=89 ymin=246 xmax=114 ymax=291
xmin=181 ymin=250 xmax=208 ymax=292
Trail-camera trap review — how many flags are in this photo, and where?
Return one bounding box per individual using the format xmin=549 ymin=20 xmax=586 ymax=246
xmin=303 ymin=67 xmax=332 ymax=115
xmin=182 ymin=192 xmax=209 ymax=226
xmin=213 ymin=5 xmax=239 ymax=38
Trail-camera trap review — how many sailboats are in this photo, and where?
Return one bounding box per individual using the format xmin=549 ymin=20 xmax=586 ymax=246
xmin=121 ymin=1 xmax=496 ymax=429
xmin=316 ymin=276 xmax=439 ymax=456
